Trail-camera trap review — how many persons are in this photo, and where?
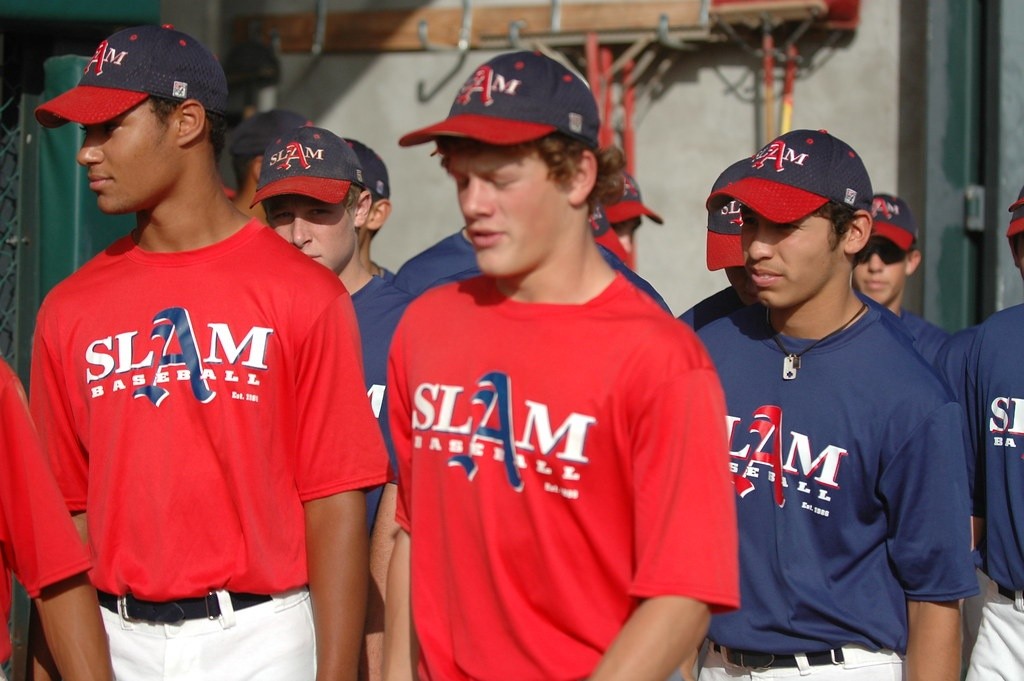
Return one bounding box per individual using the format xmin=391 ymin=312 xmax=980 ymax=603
xmin=244 ymin=129 xmax=959 ymax=681
xmin=676 ymin=129 xmax=979 ymax=681
xmin=30 ymin=24 xmax=395 ymax=681
xmin=934 ymin=185 xmax=1024 ymax=681
xmin=382 ymin=50 xmax=740 ymax=681
xmin=0 ymin=359 xmax=113 ymax=681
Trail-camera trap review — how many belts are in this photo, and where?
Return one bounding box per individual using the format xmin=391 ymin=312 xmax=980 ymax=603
xmin=95 ymin=576 xmax=271 ymax=625
xmin=704 ymin=640 xmax=846 ymax=679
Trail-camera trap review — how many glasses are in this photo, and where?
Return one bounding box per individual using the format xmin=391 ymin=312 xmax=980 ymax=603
xmin=854 ymin=242 xmax=909 ymax=265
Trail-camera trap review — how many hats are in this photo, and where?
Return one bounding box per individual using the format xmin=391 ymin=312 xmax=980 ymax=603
xmin=32 ymin=23 xmax=228 ymax=124
xmin=245 ymin=121 xmax=352 ymax=205
xmin=1005 ymin=187 xmax=1024 ymax=237
xmin=703 ymin=156 xmax=752 ymax=272
xmin=869 ymin=193 xmax=920 ymax=251
xmin=397 ymin=49 xmax=601 ymax=148
xmin=341 ymin=138 xmax=391 ymax=202
xmin=705 ymin=128 xmax=875 ymax=225
xmin=590 ymin=208 xmax=632 ymax=262
xmin=594 ymin=168 xmax=667 ymax=226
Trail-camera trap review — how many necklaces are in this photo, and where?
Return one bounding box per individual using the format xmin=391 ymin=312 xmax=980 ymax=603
xmin=767 ymin=303 xmax=868 ymax=379
